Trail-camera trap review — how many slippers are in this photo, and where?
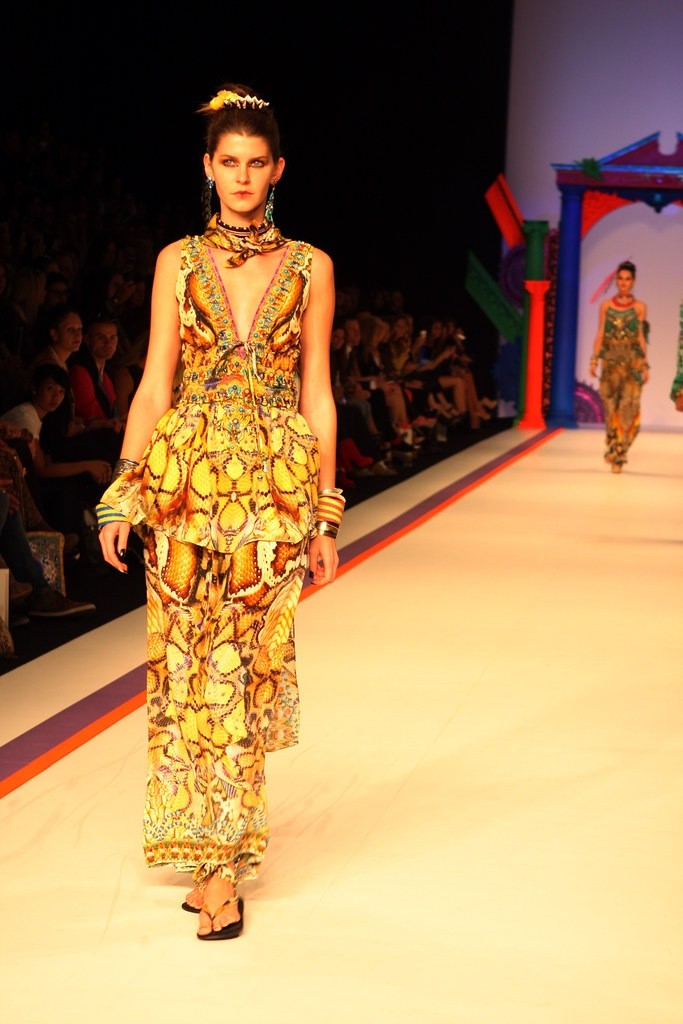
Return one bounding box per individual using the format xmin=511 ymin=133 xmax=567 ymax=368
xmin=182 ymin=881 xmax=208 ymax=913
xmin=197 ymin=893 xmax=243 ymax=940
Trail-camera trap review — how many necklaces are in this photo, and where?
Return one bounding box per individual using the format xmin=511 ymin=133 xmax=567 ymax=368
xmin=612 ymin=292 xmax=635 ymax=308
xmin=203 ymin=212 xmax=284 ymax=267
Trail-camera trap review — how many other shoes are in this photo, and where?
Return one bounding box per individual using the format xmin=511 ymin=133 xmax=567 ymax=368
xmin=612 ymin=463 xmax=622 ymax=473
xmin=46 ymin=527 xmax=79 ymax=554
xmin=469 ymin=415 xmax=481 ymax=435
xmin=480 ymin=399 xmax=497 ymax=409
xmin=476 ymin=412 xmax=491 ymax=419
xmin=437 ymin=424 xmax=446 ymax=441
xmin=447 ymin=404 xmax=464 ymax=425
xmin=372 ymin=460 xmax=399 ymax=475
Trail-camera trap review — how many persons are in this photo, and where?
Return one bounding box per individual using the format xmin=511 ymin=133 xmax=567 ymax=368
xmin=590 ymin=261 xmax=650 ymax=473
xmin=332 ymin=279 xmax=500 ymax=483
xmin=0 ymin=135 xmax=164 ymax=656
xmin=94 ymin=88 xmax=346 ymax=939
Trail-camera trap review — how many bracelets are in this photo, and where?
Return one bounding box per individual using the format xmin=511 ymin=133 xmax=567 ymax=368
xmin=313 ymin=491 xmax=344 ymax=538
xmin=111 ymin=458 xmax=138 ymax=494
xmin=590 ymin=356 xmax=598 ymax=366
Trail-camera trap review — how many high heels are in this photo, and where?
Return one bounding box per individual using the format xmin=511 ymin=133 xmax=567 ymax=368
xmin=340 ymin=438 xmax=373 ymax=471
xmin=336 ymin=462 xmax=354 ymax=488
xmin=412 ymin=418 xmax=436 ymax=435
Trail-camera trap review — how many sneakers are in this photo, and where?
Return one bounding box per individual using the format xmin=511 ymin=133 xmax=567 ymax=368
xmin=8 ymin=614 xmax=29 ymax=626
xmin=25 ymin=589 xmax=96 ymax=616
